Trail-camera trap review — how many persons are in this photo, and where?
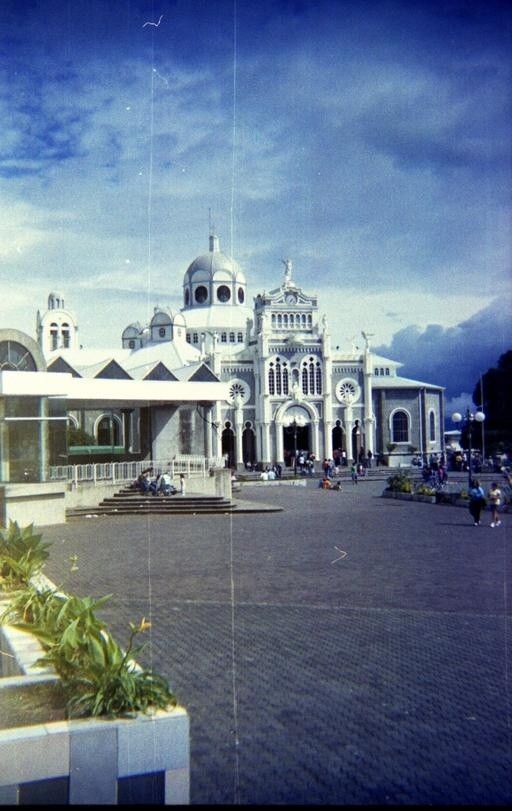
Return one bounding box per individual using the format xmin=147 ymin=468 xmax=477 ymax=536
xmin=280 ymin=257 xmax=294 ymax=290
xmin=486 ymin=483 xmax=503 ymax=529
xmin=179 ymin=474 xmax=186 ymax=497
xmin=321 ymin=313 xmax=327 ymax=334
xmin=223 ymin=449 xmax=511 ymax=495
xmin=128 ymin=468 xmax=177 ymax=497
xmin=466 ymin=479 xmax=488 ymax=527
xmin=245 ymin=317 xmax=253 ymax=345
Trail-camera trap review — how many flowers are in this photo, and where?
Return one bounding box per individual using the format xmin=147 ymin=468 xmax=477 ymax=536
xmin=31 ymin=606 xmax=180 ymax=718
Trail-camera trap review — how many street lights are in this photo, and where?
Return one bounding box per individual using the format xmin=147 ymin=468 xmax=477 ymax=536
xmin=283 ymin=418 xmax=305 ymax=478
xmin=452 ymin=405 xmax=487 ymax=489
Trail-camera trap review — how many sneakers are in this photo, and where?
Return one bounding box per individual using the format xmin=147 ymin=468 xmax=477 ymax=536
xmin=473 ymin=520 xmax=501 ymax=528
